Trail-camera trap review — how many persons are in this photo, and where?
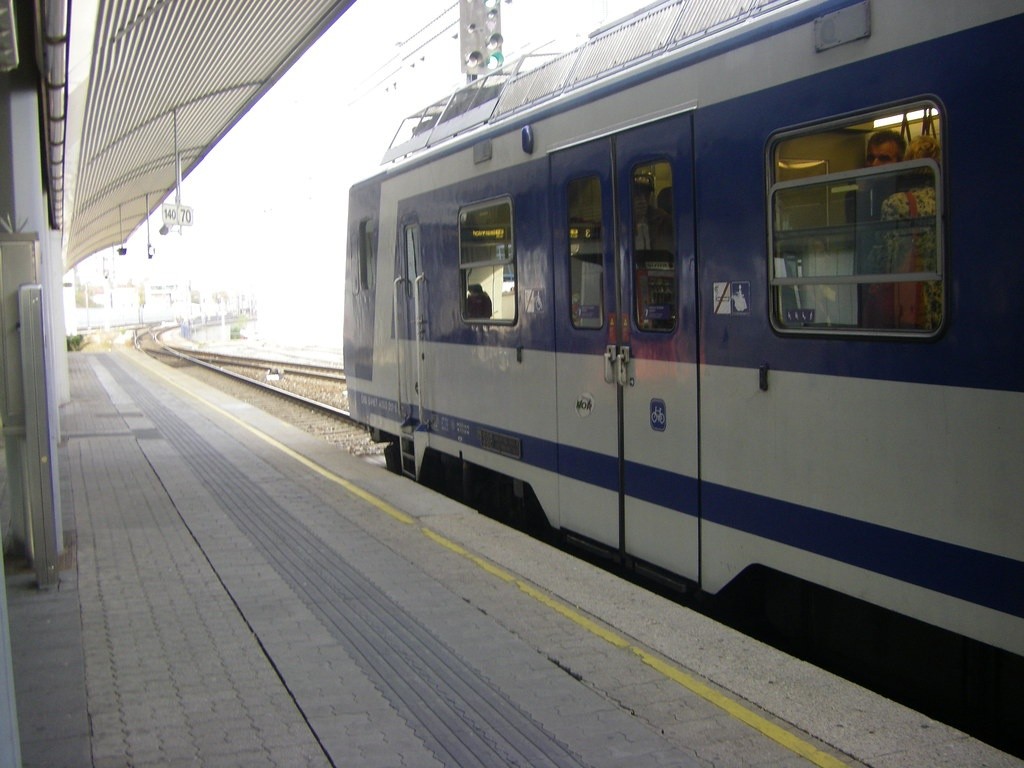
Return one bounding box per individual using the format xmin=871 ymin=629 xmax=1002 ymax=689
xmin=466 ymin=284 xmax=493 ymax=320
xmin=847 ymin=125 xmax=944 ymax=335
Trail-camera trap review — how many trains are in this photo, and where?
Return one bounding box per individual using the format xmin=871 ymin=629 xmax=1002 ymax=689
xmin=340 ymin=0 xmax=1024 ymax=682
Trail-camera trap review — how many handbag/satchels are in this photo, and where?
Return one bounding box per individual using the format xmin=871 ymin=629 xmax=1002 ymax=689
xmin=875 ymin=190 xmax=926 ymax=328
